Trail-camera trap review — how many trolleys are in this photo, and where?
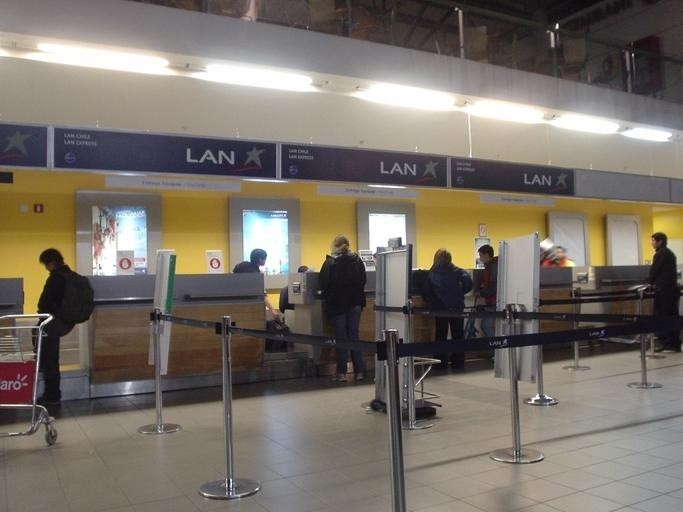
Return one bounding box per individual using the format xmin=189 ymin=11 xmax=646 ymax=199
xmin=0 ymin=312 xmax=59 ymax=446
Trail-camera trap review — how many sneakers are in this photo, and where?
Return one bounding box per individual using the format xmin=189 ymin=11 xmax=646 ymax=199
xmin=355 ymin=372 xmax=366 ymax=381
xmin=36 ymin=397 xmax=60 ymax=405
xmin=331 ymin=373 xmax=348 ymax=382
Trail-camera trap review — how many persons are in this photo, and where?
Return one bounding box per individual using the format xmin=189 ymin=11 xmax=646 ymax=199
xmin=646 ymin=231 xmax=682 ymax=355
xmin=232 ymin=249 xmax=280 ymax=325
xmin=475 ymin=244 xmax=499 ymax=357
xmin=318 ymin=234 xmax=367 ymax=381
xmin=420 ymin=247 xmax=473 ymax=372
xmin=29 ymin=246 xmax=96 ymax=408
xmin=538 ymin=246 xmax=576 ymax=267
xmin=279 ymin=266 xmax=313 ymax=312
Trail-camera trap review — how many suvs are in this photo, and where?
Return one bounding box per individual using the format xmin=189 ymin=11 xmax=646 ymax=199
xmin=134 ymin=256 xmax=146 ymax=273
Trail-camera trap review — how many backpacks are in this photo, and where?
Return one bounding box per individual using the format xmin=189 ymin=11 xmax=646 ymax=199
xmin=51 ymin=271 xmax=94 ymax=324
